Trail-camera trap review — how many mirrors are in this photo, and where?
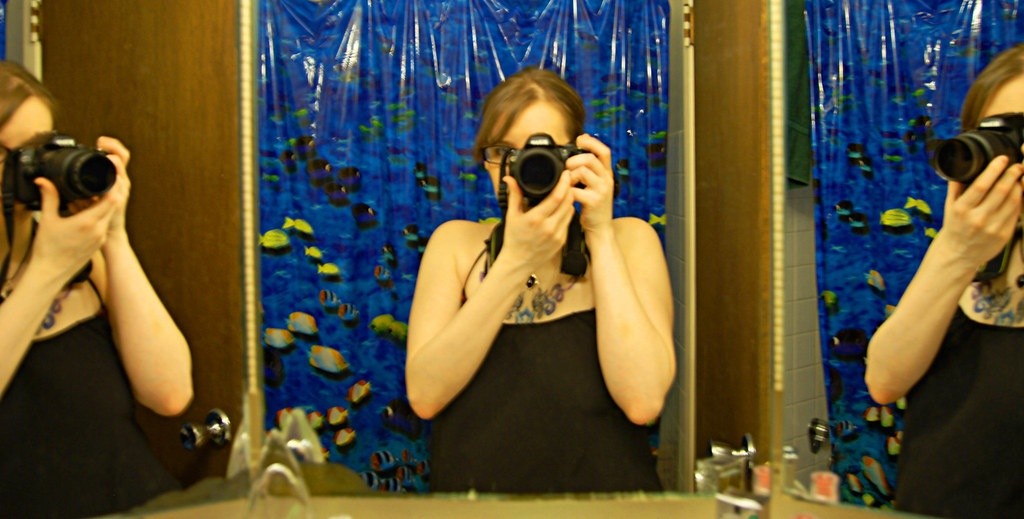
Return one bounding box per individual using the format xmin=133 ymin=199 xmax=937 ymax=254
xmin=0 ymin=0 xmax=1023 ymax=518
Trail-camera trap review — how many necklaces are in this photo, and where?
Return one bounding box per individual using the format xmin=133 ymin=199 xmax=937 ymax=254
xmin=0 ymin=240 xmax=32 ymax=299
xmin=525 ymin=274 xmax=538 ymax=290
xmin=1016 ymin=237 xmax=1024 ymax=288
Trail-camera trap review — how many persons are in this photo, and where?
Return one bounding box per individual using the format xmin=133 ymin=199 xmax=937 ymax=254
xmin=0 ymin=58 xmax=196 ymax=519
xmin=405 ymin=65 xmax=682 ymax=498
xmin=865 ymin=43 xmax=1024 ymax=519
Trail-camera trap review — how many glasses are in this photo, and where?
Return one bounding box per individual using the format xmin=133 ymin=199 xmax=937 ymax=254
xmin=480 ymin=144 xmax=512 ymax=165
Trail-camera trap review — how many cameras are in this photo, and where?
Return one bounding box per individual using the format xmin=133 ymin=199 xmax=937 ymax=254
xmin=511 ymin=134 xmax=585 ymax=204
xmin=934 ymin=113 xmax=1024 ymax=189
xmin=2 ymin=131 xmax=118 ymax=212
